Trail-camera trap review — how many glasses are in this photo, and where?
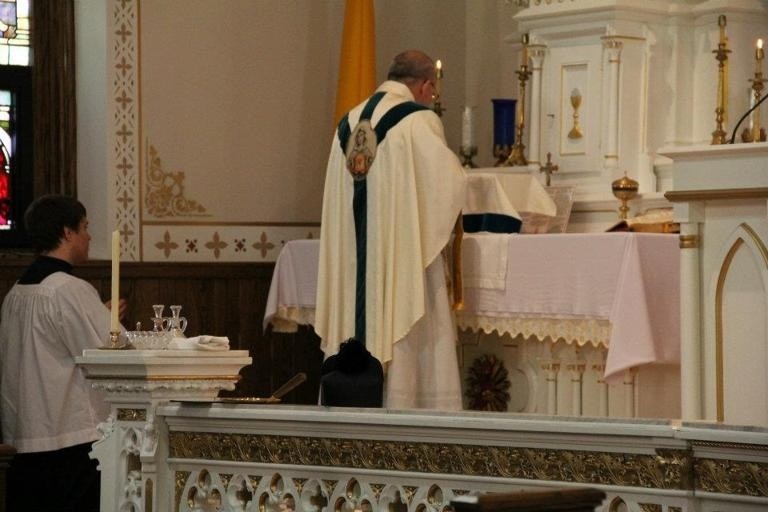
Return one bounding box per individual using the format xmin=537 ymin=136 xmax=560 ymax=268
xmin=424 ymin=79 xmax=439 ymax=101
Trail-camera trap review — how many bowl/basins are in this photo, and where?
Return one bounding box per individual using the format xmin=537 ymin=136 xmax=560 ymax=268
xmin=123 ymin=329 xmax=178 ymax=352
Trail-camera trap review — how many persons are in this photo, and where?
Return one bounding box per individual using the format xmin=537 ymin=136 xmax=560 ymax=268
xmin=347 ymin=126 xmax=374 ymax=179
xmin=0 ymin=190 xmax=127 ymax=512
xmin=313 ymin=47 xmax=468 ymax=410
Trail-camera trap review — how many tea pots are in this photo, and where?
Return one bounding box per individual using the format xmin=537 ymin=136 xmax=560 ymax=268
xmin=149 ymin=304 xmax=187 ymax=347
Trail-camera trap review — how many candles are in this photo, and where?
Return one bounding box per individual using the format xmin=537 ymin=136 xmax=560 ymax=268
xmin=433 ymin=58 xmax=443 ymax=93
xmin=460 ymin=104 xmax=471 ymax=146
xmin=753 ymin=37 xmax=765 ymax=78
xmin=109 ymin=229 xmax=121 ymax=332
